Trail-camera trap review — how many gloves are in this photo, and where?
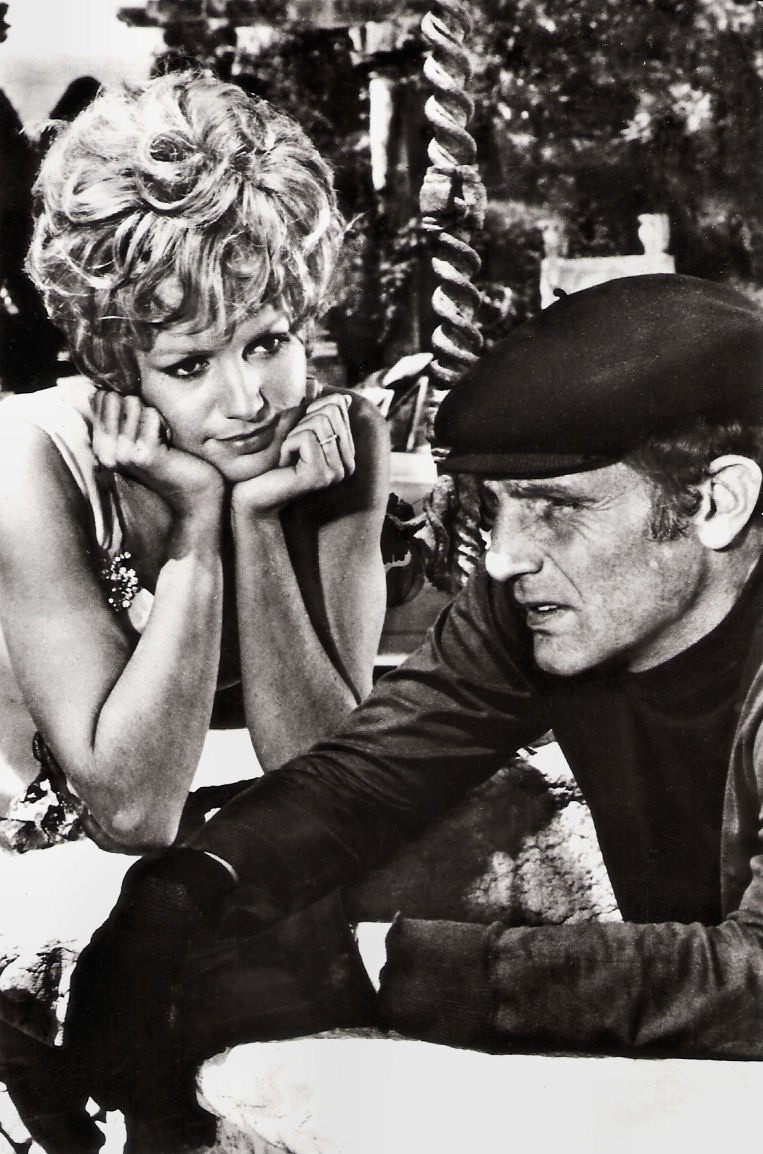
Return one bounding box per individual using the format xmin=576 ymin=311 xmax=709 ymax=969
xmin=132 ymin=884 xmax=378 ymax=1148
xmin=61 ymin=846 xmax=234 ymax=1111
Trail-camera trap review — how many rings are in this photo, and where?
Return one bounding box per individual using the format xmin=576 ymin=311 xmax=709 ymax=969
xmin=320 ymin=434 xmax=338 ymax=446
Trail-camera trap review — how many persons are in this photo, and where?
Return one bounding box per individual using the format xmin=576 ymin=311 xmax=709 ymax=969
xmin=0 ymin=273 xmax=763 ymax=1154
xmin=0 ymin=68 xmax=391 ymax=851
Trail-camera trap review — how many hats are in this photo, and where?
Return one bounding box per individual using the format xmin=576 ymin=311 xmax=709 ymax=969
xmin=434 ymin=273 xmax=763 ymax=479
